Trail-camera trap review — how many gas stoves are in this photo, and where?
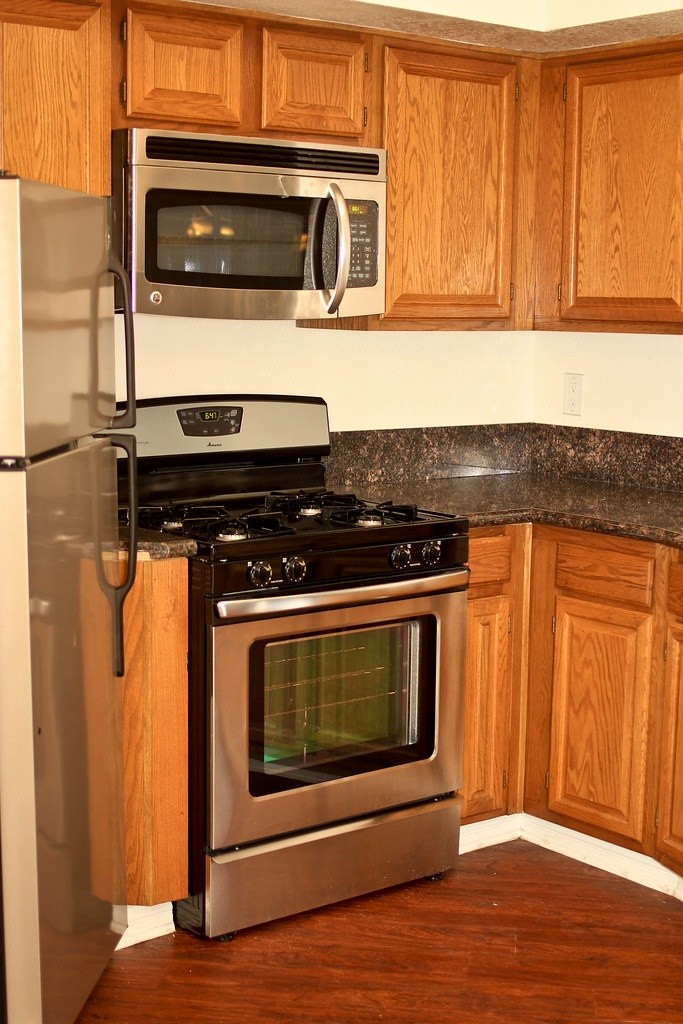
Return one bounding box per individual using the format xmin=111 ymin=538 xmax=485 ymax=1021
xmin=113 ymin=393 xmax=473 ymax=596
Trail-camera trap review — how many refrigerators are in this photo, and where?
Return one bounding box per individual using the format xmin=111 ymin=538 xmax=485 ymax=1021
xmin=2 ymin=172 xmax=141 ymax=1024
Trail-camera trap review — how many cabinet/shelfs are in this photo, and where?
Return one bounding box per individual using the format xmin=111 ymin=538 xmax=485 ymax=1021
xmin=1 ymin=0 xmax=112 ymax=198
xmin=541 ymin=35 xmax=683 ymax=334
xmin=111 ymin=1 xmax=377 ymax=330
xmin=383 ymin=30 xmax=542 ymax=331
xmin=656 ymin=546 xmax=682 ymax=878
xmin=522 ymin=522 xmax=667 ymax=859
xmin=77 ymin=523 xmax=188 ymax=907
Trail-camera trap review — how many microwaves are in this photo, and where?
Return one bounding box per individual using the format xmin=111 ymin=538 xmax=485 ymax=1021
xmin=112 ymin=127 xmax=387 ymax=323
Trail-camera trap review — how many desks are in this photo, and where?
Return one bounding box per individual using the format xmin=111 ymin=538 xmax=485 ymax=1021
xmin=459 ymin=524 xmax=530 ymax=827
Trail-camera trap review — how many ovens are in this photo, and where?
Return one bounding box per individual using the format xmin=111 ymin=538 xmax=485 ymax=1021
xmin=170 ymin=590 xmax=470 ymax=943
